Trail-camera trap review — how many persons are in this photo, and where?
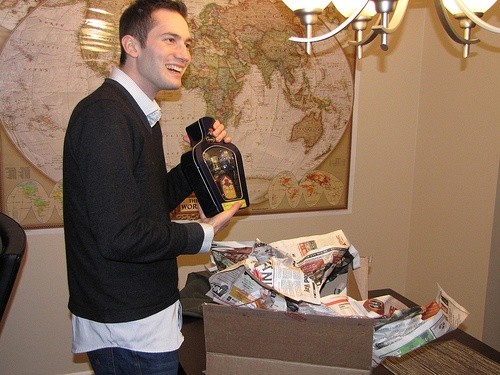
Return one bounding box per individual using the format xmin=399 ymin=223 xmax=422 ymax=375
xmin=63 ymin=0 xmax=244 ymax=375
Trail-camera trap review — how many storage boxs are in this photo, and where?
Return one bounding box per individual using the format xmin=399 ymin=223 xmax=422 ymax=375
xmin=202 ymin=303 xmax=374 ymax=375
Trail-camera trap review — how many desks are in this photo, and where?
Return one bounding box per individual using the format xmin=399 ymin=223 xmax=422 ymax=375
xmin=178 ymin=288 xmax=500 ymax=375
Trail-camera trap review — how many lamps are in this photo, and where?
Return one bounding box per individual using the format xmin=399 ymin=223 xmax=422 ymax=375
xmin=279 ymin=0 xmax=500 ymax=57
